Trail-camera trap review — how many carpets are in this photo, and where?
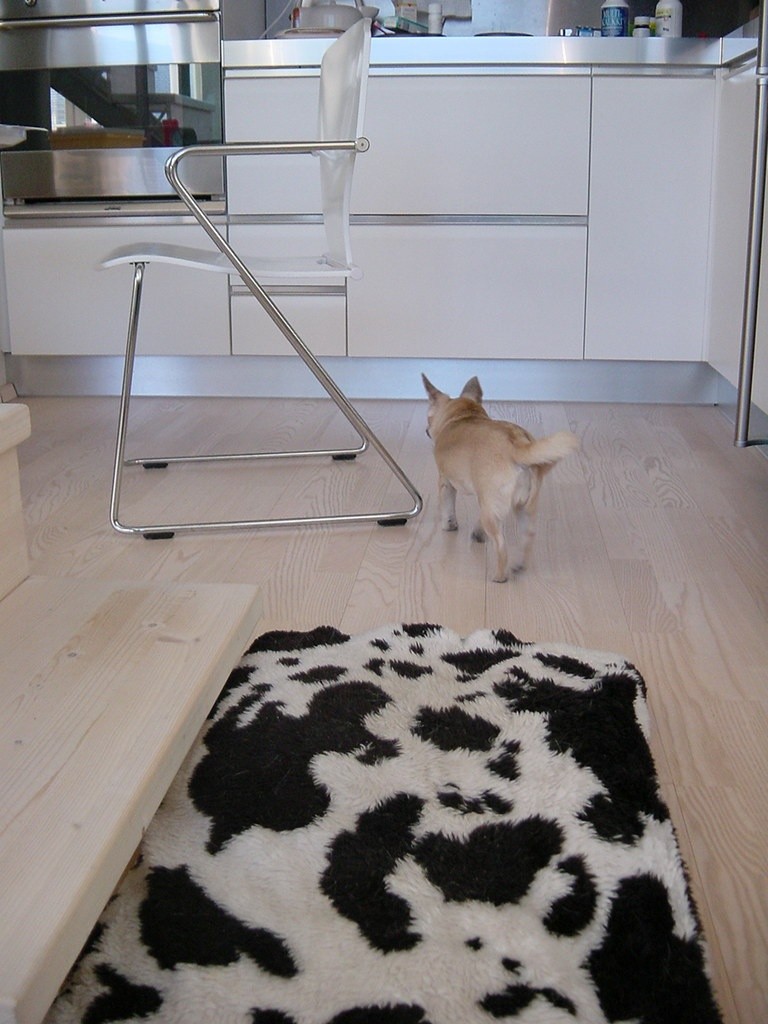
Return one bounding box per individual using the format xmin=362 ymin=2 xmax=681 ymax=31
xmin=44 ymin=622 xmax=725 ymax=1024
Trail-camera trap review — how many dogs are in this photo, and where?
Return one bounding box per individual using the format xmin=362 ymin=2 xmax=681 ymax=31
xmin=422 ymin=372 xmax=575 ymax=583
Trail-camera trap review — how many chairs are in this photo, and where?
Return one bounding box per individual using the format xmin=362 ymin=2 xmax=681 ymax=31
xmin=97 ymin=16 xmax=424 ymax=542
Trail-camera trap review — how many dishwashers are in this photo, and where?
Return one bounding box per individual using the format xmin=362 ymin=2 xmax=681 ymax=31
xmin=0 ymin=11 xmax=226 ymax=202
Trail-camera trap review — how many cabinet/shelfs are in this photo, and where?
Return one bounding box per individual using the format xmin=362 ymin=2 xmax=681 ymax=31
xmin=221 ymin=67 xmax=594 ymax=404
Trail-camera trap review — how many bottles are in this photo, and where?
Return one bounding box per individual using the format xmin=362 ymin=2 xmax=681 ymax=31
xmin=654 ymin=0 xmax=684 ymax=38
xmin=600 ymin=0 xmax=630 ymax=38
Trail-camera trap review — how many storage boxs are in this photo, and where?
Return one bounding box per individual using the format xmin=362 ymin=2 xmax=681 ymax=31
xmin=381 ymin=16 xmax=428 ymax=34
xmin=395 ymin=0 xmax=418 ymax=23
